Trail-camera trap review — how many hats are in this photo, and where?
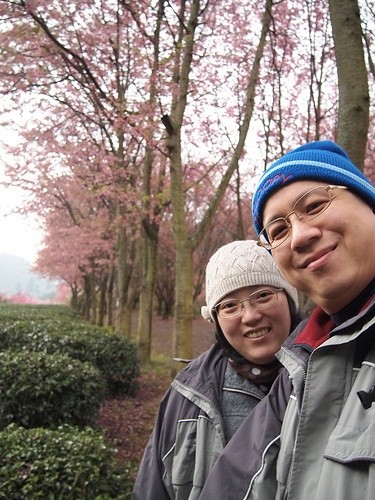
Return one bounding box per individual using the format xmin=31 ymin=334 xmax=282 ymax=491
xmin=251 ymin=141 xmax=375 ymax=256
xmin=202 ymin=241 xmax=299 ymax=324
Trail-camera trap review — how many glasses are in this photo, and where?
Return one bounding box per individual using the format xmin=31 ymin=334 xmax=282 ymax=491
xmin=257 ymin=185 xmax=349 ymax=250
xmin=211 ymin=288 xmax=282 ymax=319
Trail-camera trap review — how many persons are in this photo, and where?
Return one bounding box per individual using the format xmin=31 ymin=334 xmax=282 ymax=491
xmin=129 ymin=238 xmax=299 ymax=500
xmin=200 ymin=138 xmax=375 ymax=500
